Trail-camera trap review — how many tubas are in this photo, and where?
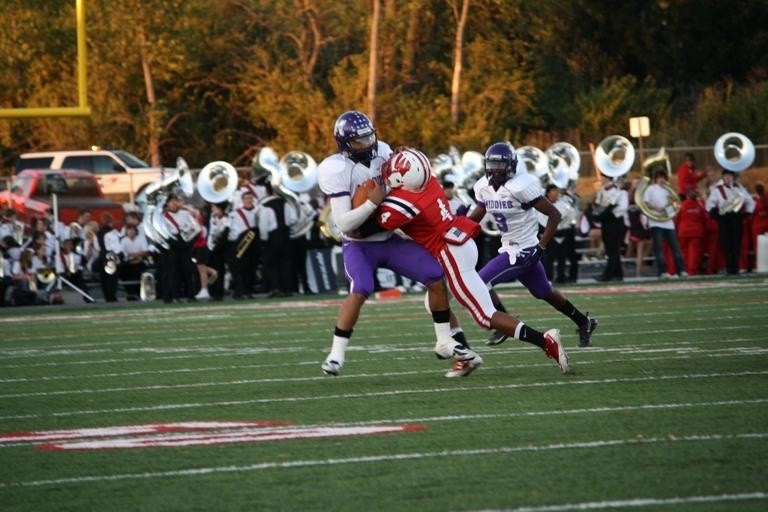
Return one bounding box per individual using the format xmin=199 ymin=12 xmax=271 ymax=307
xmin=144 ymin=143 xmax=319 ymax=239
xmin=707 ymin=133 xmax=755 ymax=217
xmin=437 ymin=142 xmax=580 ymax=227
xmin=635 ymin=147 xmax=681 ymax=222
xmin=592 ymin=135 xmax=635 ymax=214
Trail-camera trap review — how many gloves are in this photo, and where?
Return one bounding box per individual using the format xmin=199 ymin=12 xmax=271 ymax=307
xmin=523 ymin=243 xmax=548 ymax=268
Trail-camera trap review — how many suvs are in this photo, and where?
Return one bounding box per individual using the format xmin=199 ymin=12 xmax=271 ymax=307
xmin=7 ymin=146 xmax=200 ymax=199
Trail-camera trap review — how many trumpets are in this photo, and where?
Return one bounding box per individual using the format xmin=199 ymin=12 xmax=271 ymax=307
xmin=235 ymin=230 xmax=254 ymax=259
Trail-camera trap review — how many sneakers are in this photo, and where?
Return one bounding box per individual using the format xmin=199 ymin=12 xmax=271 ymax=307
xmin=433 ymin=339 xmax=471 ymax=360
xmin=548 ymin=267 xmax=690 ymax=286
xmin=444 ymin=351 xmax=483 ymax=380
xmin=576 ymin=314 xmax=600 ymax=349
xmin=320 ymin=350 xmax=343 ymax=376
xmin=194 ymin=287 xmax=211 ymax=299
xmin=541 ymin=326 xmax=569 ymax=375
xmin=484 ymin=327 xmax=513 ymax=347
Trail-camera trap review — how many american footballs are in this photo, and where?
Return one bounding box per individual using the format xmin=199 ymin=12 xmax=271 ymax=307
xmin=354 ymin=180 xmax=376 ymax=208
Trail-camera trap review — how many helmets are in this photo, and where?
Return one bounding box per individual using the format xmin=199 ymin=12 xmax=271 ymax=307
xmin=382 ymin=148 xmax=431 ymax=194
xmin=484 ymin=142 xmax=519 ymax=175
xmin=333 ymin=112 xmax=380 ymax=155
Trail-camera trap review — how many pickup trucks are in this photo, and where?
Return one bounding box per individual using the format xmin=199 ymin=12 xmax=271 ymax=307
xmin=0 ymin=169 xmax=126 ymax=239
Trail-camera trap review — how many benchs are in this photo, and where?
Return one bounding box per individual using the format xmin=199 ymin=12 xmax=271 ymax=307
xmin=573 ymin=231 xmax=661 ymax=282
